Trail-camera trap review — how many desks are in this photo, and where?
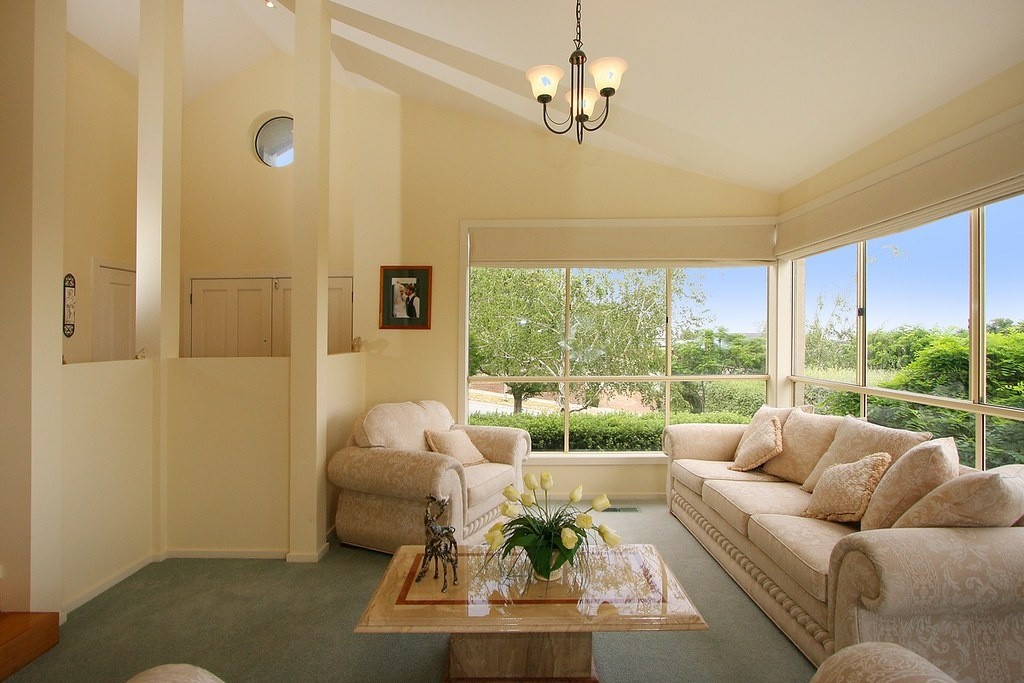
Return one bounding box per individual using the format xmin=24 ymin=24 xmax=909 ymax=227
xmin=353 ymin=544 xmax=709 ymax=683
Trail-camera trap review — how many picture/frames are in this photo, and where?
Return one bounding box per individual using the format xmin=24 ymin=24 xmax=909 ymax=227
xmin=379 ymin=266 xmax=433 ymax=330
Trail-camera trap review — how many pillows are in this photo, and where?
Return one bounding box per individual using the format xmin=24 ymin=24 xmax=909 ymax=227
xmin=727 ymin=416 xmax=783 ymax=471
xmin=801 ymin=452 xmax=892 ymax=522
xmin=424 ymin=429 xmax=489 ymax=467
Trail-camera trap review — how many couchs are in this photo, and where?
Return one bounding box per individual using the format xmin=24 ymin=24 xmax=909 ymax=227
xmin=661 ymin=404 xmax=1024 ymax=683
xmin=327 ymin=399 xmax=531 ymax=554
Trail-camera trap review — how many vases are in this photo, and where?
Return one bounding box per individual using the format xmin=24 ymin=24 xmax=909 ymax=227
xmin=533 ymin=550 xmax=563 ymax=581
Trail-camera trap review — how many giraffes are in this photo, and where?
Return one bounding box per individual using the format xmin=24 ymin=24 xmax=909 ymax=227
xmin=415 ymin=493 xmax=459 ymax=592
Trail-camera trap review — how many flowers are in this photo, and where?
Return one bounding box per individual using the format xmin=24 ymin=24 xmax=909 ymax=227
xmin=481 ymin=470 xmax=621 ymax=570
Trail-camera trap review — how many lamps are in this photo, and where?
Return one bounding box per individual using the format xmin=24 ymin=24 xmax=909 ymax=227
xmin=525 ymin=0 xmax=627 ymax=144
